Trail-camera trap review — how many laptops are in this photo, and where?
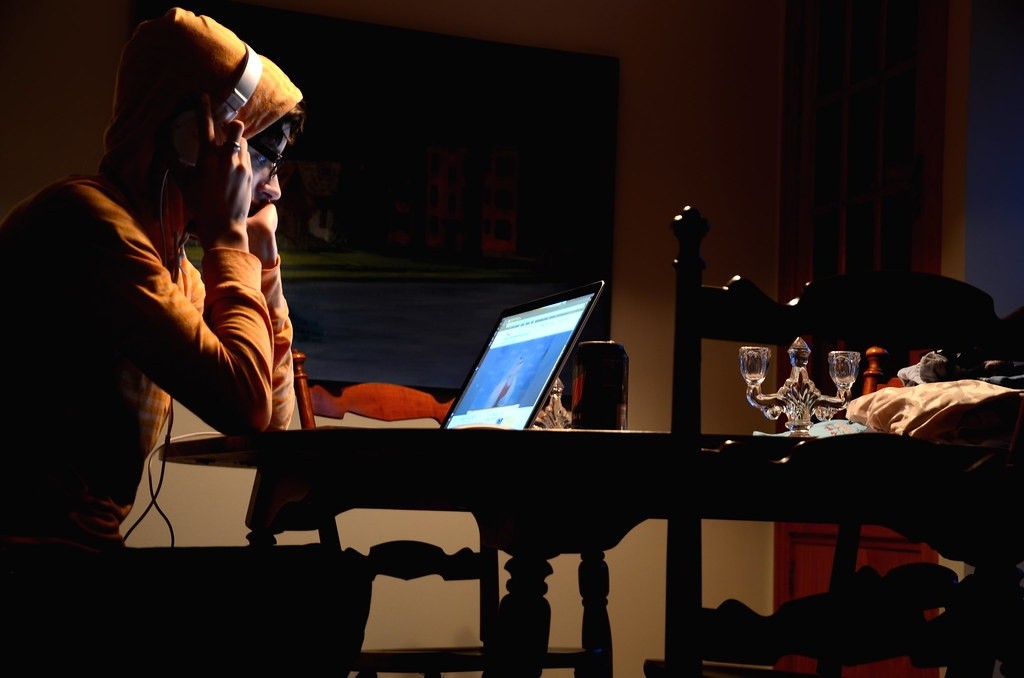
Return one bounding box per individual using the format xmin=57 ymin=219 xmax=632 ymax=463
xmin=440 ymin=280 xmax=605 ymax=430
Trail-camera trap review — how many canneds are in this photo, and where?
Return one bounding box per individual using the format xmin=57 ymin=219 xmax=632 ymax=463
xmin=572 ymin=340 xmax=628 ymax=430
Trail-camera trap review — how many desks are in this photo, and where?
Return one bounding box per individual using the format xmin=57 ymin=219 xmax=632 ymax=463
xmin=154 ymin=424 xmax=1024 ymax=678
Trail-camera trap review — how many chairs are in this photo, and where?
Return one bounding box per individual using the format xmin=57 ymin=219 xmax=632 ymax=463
xmin=643 ymin=204 xmax=1024 ymax=678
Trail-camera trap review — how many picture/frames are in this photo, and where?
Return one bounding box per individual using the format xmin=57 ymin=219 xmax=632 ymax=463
xmin=128 ymin=0 xmax=621 ymax=415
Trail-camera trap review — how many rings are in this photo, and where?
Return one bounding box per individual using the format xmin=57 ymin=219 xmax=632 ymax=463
xmin=223 ymin=139 xmax=238 ymax=148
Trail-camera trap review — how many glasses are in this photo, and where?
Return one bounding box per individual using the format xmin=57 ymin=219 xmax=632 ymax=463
xmin=247 ymin=139 xmax=286 ymax=180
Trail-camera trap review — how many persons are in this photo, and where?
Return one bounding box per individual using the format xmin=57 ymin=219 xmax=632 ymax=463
xmin=485 ymin=358 xmax=523 ymax=408
xmin=0 ymin=7 xmax=372 ymax=678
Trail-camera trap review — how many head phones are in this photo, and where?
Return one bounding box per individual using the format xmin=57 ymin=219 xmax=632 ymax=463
xmin=170 ymin=41 xmax=263 ymax=166
